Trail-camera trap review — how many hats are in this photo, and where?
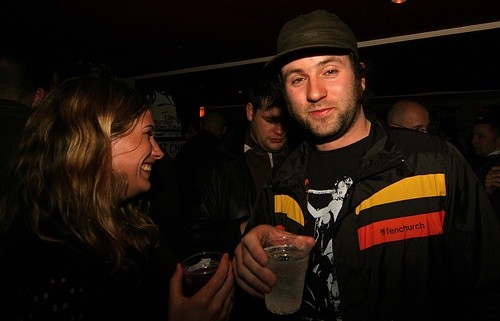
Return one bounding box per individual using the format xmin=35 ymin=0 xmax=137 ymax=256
xmin=263 ymin=9 xmax=359 ymax=73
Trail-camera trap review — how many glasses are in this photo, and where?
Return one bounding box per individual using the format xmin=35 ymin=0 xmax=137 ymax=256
xmin=394 ymin=123 xmax=433 ymax=133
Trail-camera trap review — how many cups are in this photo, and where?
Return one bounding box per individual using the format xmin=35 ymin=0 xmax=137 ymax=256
xmin=181 ymin=252 xmax=223 ymax=298
xmin=262 ymin=235 xmax=312 ymax=315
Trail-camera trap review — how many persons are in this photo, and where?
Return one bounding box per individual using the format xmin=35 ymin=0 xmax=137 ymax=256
xmin=0 ymin=64 xmax=237 ymax=321
xmin=0 ymin=56 xmax=45 ymax=168
xmin=476 ymin=150 xmax=500 ymax=222
xmin=387 ymin=98 xmax=430 ymax=134
xmin=472 ymin=103 xmax=498 ymax=154
xmin=231 ymin=9 xmax=500 ymax=321
xmin=174 ymin=72 xmax=293 ymax=248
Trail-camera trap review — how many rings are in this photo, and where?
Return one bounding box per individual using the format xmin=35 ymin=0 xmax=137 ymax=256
xmin=228 ymin=294 xmax=235 ymax=301
